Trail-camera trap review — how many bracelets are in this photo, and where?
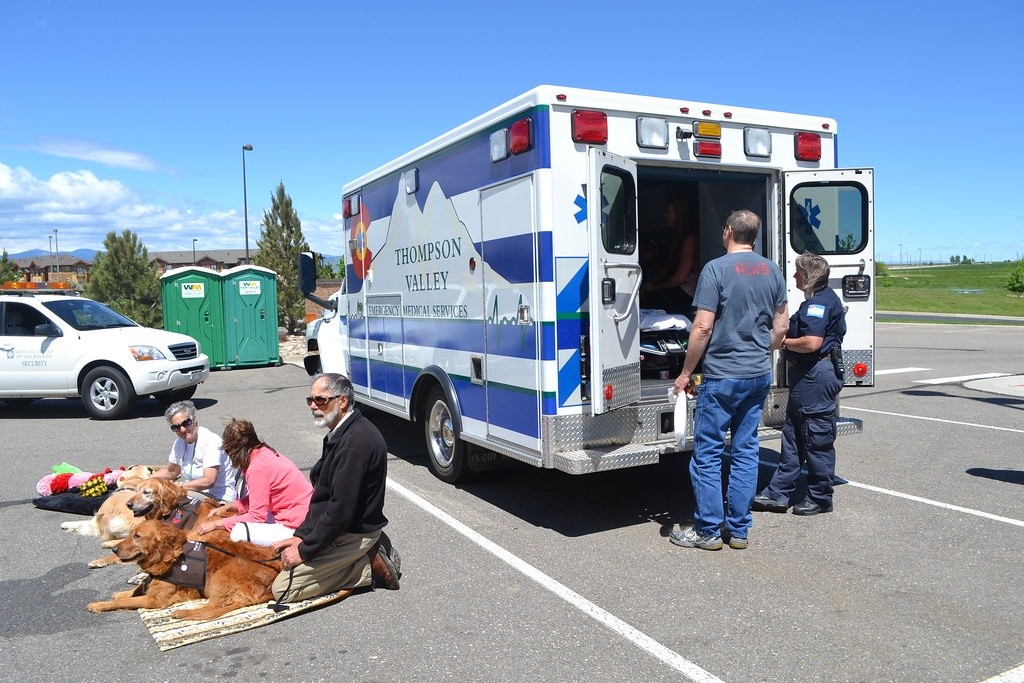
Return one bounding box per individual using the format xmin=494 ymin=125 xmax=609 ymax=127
xmin=782 ymin=340 xmax=787 ymax=350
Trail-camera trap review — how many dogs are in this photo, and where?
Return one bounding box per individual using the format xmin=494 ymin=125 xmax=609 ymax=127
xmin=85 ymin=520 xmax=284 ymax=622
xmin=62 ymin=476 xmax=145 ymax=543
xmin=101 ymin=477 xmax=236 ymax=549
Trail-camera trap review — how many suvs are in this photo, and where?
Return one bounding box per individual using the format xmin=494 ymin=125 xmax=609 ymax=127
xmin=0 ymin=290 xmax=210 ymax=422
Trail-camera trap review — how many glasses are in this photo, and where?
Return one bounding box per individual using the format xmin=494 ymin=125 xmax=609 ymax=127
xmin=721 ymin=225 xmax=729 ymax=231
xmin=307 ymin=394 xmax=344 ymax=407
xmin=171 ymin=418 xmax=193 ymax=431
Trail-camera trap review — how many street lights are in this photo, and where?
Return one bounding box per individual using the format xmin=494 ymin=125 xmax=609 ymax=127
xmin=918 ymin=248 xmax=921 ymax=276
xmin=53 ymin=229 xmax=60 ymax=283
xmin=48 ymin=236 xmax=54 ymax=283
xmin=193 ymin=238 xmax=197 ymax=267
xmin=242 ymin=143 xmax=254 ymax=265
xmin=898 ymin=244 xmax=902 ymax=275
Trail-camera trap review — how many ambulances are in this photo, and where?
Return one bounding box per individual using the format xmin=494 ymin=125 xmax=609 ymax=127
xmin=297 ymin=84 xmax=876 ymax=487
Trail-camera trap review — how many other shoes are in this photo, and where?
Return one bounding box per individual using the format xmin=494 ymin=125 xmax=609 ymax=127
xmin=374 ymin=530 xmax=401 ymax=578
xmin=366 ymin=544 xmax=399 ymax=590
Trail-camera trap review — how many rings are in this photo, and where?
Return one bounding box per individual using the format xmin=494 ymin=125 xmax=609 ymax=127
xmin=284 ymin=563 xmax=287 ymax=566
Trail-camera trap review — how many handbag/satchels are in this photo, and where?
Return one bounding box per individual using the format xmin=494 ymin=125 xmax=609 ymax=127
xmin=668 ymin=387 xmax=687 ymax=450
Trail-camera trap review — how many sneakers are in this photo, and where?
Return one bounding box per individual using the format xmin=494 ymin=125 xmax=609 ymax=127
xmin=670 ymin=523 xmax=723 ymax=550
xmin=720 ymin=526 xmax=746 ymax=550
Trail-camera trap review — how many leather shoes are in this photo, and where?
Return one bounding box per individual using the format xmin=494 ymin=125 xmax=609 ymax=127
xmin=793 ymin=499 xmax=833 ymax=514
xmin=750 ymin=491 xmax=790 ymax=514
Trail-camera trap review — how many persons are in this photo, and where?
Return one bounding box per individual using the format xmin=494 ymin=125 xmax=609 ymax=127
xmin=642 ymin=202 xmax=700 ymax=316
xmin=670 ymin=210 xmax=789 ymax=549
xmin=639 ymin=238 xmax=663 ymax=298
xmin=152 ymin=400 xmax=238 ymax=506
xmin=196 ymin=420 xmax=315 ymax=547
xmin=271 ymin=373 xmax=401 ymax=603
xmin=755 ymin=252 xmax=846 ymax=516
